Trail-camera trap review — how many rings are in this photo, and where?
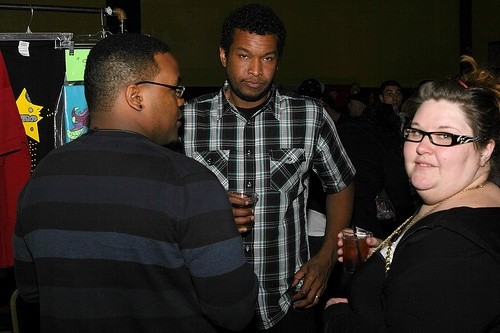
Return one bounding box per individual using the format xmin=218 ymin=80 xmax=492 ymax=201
xmin=315 ymin=296 xmax=320 ymax=299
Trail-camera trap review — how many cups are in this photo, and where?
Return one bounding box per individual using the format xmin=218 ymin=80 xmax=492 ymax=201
xmin=226 ymin=189 xmax=258 ymax=227
xmin=342 ymin=230 xmax=372 ymax=274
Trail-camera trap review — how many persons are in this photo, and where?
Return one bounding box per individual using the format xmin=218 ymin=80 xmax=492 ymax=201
xmin=14 ymin=32 xmax=258 ymax=333
xmin=166 ymin=3 xmax=356 ymax=333
xmin=294 ymin=78 xmax=423 ymax=236
xmin=322 ymin=55 xmax=500 ymax=333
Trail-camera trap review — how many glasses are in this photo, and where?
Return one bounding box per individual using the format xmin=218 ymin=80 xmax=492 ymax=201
xmin=135 ymin=79 xmax=186 ymax=99
xmin=386 ymin=92 xmax=399 ymax=96
xmin=403 ymin=128 xmax=475 ymax=147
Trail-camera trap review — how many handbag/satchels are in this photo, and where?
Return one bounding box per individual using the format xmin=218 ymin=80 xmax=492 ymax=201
xmin=375 ymin=191 xmax=396 ymax=221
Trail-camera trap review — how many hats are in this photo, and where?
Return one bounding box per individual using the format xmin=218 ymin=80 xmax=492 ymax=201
xmin=350 ymin=92 xmax=369 ymax=106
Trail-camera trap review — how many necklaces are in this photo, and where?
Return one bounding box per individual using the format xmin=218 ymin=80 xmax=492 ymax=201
xmin=367 ymin=180 xmax=489 ymax=271
